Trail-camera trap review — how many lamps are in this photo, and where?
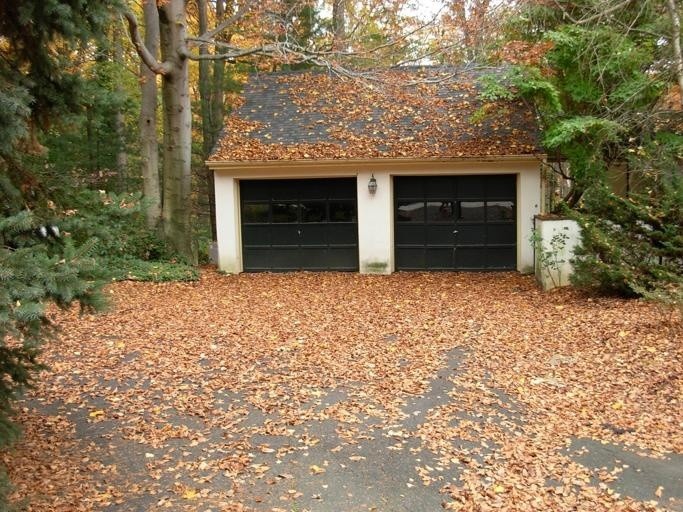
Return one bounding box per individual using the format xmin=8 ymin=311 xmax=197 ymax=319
xmin=367 ymin=173 xmax=378 ymax=195
